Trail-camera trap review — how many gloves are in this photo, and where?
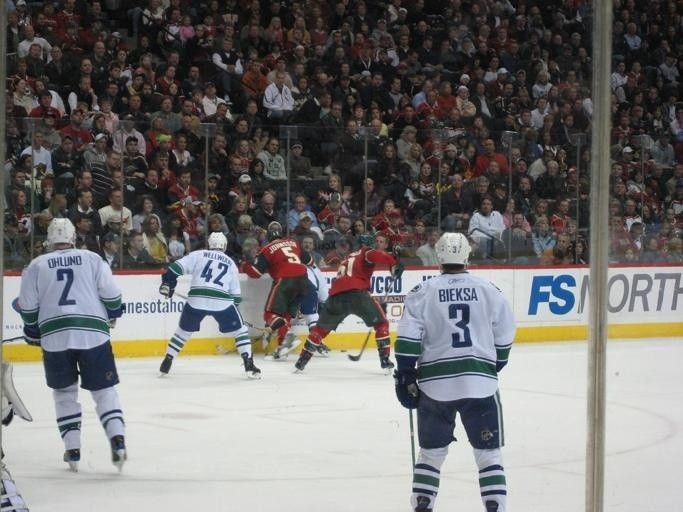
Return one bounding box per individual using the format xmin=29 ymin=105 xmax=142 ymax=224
xmin=389 ymin=262 xmax=404 ymax=282
xmin=157 ymin=275 xmax=177 ymax=301
xmin=391 ymin=367 xmax=421 ymax=410
xmin=21 ymin=323 xmax=41 ymax=348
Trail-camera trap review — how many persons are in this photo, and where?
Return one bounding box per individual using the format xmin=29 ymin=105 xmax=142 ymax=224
xmin=98 ymin=190 xmax=131 ymax=231
xmin=6 ymin=68 xmax=118 ymax=151
xmin=90 ymin=150 xmax=122 ymax=192
xmin=371 ymin=199 xmax=402 ymax=228
xmin=405 ymin=222 xmax=426 ymax=256
xmin=256 ymin=139 xmax=288 ymax=180
xmin=320 ymin=192 xmax=350 ymax=225
xmin=5 ymin=168 xmax=174 ymax=213
xmin=240 ymin=221 xmax=332 ymax=358
xmin=394 ymin=233 xmax=516 ymax=512
xmin=54 ymin=135 xmax=86 ymax=179
xmin=38 ymin=193 xmax=69 ymax=230
xmin=378 ymin=210 xmax=407 ymax=247
xmin=293 ymin=235 xmax=399 ymax=374
xmin=323 ymin=237 xmax=353 ymax=268
xmin=92 ymin=232 xmax=125 ymax=270
xmin=203 ymin=69 xmax=374 ymax=167
xmin=134 ymin=196 xmax=163 ymax=235
xmin=13 ymin=218 xmax=130 ymax=472
xmin=114 ymin=69 xmax=205 ymax=167
xmin=4 ymin=187 xmax=38 ymax=231
xmin=123 ymin=230 xmax=159 ymax=270
xmin=291 ymin=169 xmax=507 ymax=233
xmin=82 ymin=133 xmax=108 ymax=172
xmin=200 ymin=167 xmax=274 ymax=235
xmin=254 ymin=191 xmax=284 ymax=229
xmin=21 ymin=130 xmax=52 ymax=178
xmin=157 ymin=233 xmax=265 ymax=378
xmin=4 ymin=214 xmax=32 ymax=271
xmin=505 ymin=1 xmax=682 ymax=265
xmin=167 ymin=166 xmax=208 ymax=242
xmin=367 ymin=235 xmax=389 ymax=253
xmin=68 ymin=190 xmax=101 ymax=231
xmin=374 ymin=69 xmax=504 ymax=173
xmin=440 ymin=174 xmax=473 ymax=233
xmin=3 ymin=141 xmax=21 ymax=172
xmin=165 ymin=214 xmax=191 ymax=261
xmin=141 ymin=216 xmax=168 ymax=263
xmin=16 ymin=239 xmax=43 ymax=271
xmin=7 ymin=1 xmax=504 ymax=68
xmin=300 ymin=236 xmax=325 ymax=267
xmin=261 ymin=261 xmax=333 ymax=357
xmin=286 ymin=195 xmax=318 ymax=235
xmin=238 ymin=237 xmax=259 ymax=270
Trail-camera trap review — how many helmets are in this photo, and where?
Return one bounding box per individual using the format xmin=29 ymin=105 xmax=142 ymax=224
xmin=434 ymin=232 xmax=473 ymax=266
xmin=358 ymin=234 xmax=376 ymax=250
xmin=206 ymin=230 xmax=228 ymax=251
xmin=266 ymin=221 xmax=283 ymax=241
xmin=44 ymin=214 xmax=79 ymax=247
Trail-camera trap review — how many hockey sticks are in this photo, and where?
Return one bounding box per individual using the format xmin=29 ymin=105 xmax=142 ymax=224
xmin=347 ymin=264 xmax=405 ymax=361
xmin=174 ymin=291 xmax=286 ymax=333
xmin=216 ymin=333 xmax=264 ymax=356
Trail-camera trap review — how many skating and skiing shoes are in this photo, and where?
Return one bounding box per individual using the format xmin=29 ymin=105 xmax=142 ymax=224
xmin=159 ymin=353 xmax=173 ymax=375
xmin=262 ymin=324 xmax=278 ymax=351
xmin=60 ymin=445 xmax=80 ymax=472
xmin=111 ymin=433 xmax=128 ymax=469
xmin=316 ymin=343 xmax=332 ymax=357
xmin=241 ymin=353 xmax=263 ymax=379
xmin=295 ymin=351 xmax=313 ymax=370
xmin=271 ymin=334 xmax=302 ymax=359
xmin=380 ymin=357 xmax=395 ymax=376
